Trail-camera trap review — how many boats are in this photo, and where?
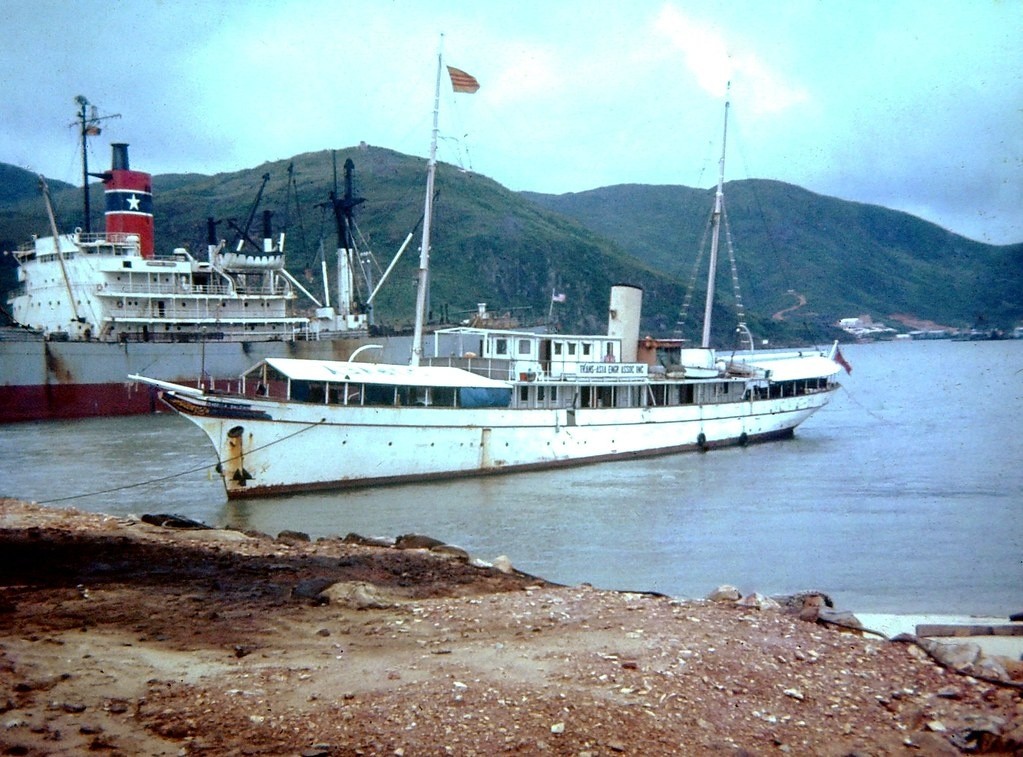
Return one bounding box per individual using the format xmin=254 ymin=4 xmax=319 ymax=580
xmin=121 ymin=26 xmax=852 ymax=499
xmin=1 ymin=94 xmax=441 ymax=426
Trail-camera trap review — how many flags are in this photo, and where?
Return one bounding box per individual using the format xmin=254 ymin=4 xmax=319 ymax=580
xmin=86 ymin=125 xmax=101 ymax=135
xmin=833 ymin=350 xmax=852 ymax=374
xmin=447 ymin=66 xmax=479 ymax=93
